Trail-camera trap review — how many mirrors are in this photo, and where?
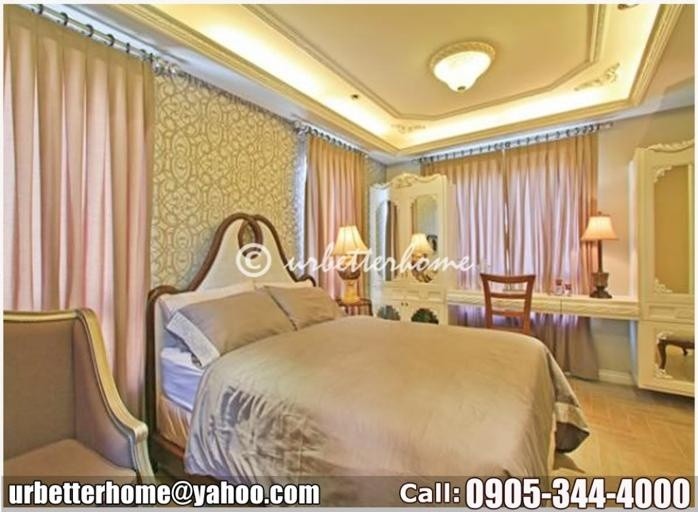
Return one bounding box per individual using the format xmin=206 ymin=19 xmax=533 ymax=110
xmin=373 ymin=199 xmax=399 ymax=282
xmin=654 ymin=333 xmax=696 ymax=382
xmin=411 ymin=195 xmax=439 ymax=282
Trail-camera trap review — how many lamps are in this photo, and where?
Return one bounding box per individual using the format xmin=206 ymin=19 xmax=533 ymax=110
xmin=579 ymin=215 xmax=619 ymax=298
xmin=330 ymin=224 xmax=369 ymax=303
xmin=412 ymin=233 xmax=434 ymax=276
xmin=429 ymin=39 xmax=496 ymax=92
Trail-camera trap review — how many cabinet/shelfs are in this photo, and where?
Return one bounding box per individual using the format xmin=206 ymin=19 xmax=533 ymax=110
xmin=629 ymin=137 xmax=695 ymax=398
xmin=367 ymin=173 xmax=455 ymax=325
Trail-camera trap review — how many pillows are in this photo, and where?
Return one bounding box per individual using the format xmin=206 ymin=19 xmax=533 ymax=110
xmin=166 ymin=289 xmax=296 ymax=366
xmin=264 ymin=285 xmax=346 ymax=331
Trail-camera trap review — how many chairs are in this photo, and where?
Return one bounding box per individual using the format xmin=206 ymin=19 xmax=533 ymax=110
xmin=479 ymin=273 xmax=537 ymax=334
xmin=659 ymin=341 xmax=693 ymax=380
xmin=5 ymin=309 xmax=158 ymax=507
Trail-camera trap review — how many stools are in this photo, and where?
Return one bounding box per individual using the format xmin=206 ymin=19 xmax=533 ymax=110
xmin=336 ymin=299 xmax=372 ymax=315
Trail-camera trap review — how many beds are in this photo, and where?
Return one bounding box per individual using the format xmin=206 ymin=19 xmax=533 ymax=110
xmin=145 ymin=212 xmax=589 ymax=504
